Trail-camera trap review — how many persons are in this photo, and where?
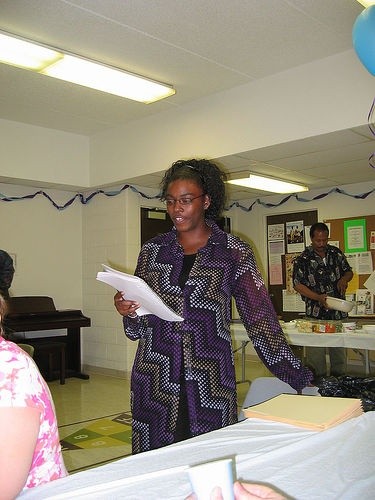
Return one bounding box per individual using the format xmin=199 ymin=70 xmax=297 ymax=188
xmin=0 ymin=249 xmax=70 ymax=500
xmin=113 ymin=159 xmax=319 ymax=454
xmin=293 ymin=223 xmax=353 ymax=377
xmin=290 ymin=225 xmax=304 ymax=243
xmin=184 ymin=480 xmax=289 ymax=500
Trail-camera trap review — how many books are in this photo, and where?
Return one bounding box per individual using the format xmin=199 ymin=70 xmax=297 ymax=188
xmin=96 ymin=264 xmax=184 ymax=322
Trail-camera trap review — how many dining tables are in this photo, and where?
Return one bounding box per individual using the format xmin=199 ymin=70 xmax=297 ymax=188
xmin=230 ymin=322 xmax=375 ymax=386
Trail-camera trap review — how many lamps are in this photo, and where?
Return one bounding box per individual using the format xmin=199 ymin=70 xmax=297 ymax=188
xmin=0 ymin=30 xmax=176 ymax=104
xmin=221 ymin=170 xmax=310 ymax=195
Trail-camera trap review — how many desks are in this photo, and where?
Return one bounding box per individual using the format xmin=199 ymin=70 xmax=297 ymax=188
xmin=15 ymin=410 xmax=375 ymax=500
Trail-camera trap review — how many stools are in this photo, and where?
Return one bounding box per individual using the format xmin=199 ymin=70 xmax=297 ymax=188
xmin=34 ymin=341 xmax=66 ymax=385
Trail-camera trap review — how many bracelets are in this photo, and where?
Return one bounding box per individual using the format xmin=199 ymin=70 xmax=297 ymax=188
xmin=128 ymin=314 xmax=137 ymax=318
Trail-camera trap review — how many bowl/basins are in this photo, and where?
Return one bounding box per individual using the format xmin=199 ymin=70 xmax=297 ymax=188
xmin=342 ymin=323 xmax=356 ymax=331
xmin=284 ymin=322 xmax=295 ymax=329
xmin=362 ymin=325 xmax=375 ymax=334
xmin=325 ymin=296 xmax=355 ymax=312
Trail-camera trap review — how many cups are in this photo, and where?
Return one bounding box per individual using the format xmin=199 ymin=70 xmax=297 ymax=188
xmin=185 ymin=458 xmax=235 ymax=500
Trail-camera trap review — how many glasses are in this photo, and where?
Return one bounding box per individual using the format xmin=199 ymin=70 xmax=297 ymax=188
xmin=162 ymin=194 xmax=205 ymax=206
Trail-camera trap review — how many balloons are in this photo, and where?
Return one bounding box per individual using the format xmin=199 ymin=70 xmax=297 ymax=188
xmin=352 ymin=4 xmax=375 ymax=77
